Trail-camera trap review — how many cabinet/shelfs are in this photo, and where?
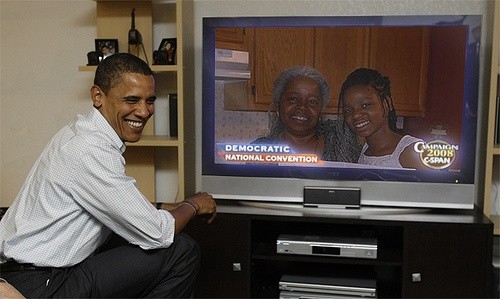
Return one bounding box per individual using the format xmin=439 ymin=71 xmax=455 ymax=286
xmin=181 ymin=204 xmax=494 ymax=299
xmin=215 ymin=27 xmax=430 ymax=119
xmin=79 ymin=0 xmax=196 ymax=210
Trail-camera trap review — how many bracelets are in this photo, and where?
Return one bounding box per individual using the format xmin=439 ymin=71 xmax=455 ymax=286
xmin=156 ymin=202 xmax=162 ymax=209
xmin=177 ymin=200 xmax=197 ymax=216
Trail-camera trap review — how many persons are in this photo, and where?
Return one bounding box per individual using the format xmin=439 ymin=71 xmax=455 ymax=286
xmin=100 ymin=46 xmax=113 ymax=60
xmin=161 ymin=42 xmax=173 ymax=61
xmin=246 ymin=65 xmax=363 ymax=165
xmin=0 ymin=53 xmax=219 ymax=299
xmin=333 ymin=65 xmax=442 ymax=171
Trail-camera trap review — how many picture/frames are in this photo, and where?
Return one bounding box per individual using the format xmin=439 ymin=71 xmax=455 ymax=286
xmin=158 ymin=37 xmax=178 ymax=64
xmin=95 ymin=38 xmax=118 ymax=60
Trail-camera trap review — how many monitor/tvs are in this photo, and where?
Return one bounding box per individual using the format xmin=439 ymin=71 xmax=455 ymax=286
xmin=192 ymin=1 xmax=489 ymax=223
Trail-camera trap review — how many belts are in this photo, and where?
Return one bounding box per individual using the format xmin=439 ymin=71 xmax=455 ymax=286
xmin=0 ymin=263 xmax=49 ymax=272
